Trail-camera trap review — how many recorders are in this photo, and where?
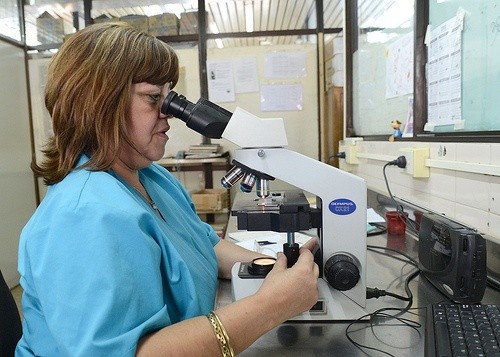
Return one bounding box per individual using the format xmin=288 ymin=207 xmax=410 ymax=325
xmin=418 ymin=211 xmax=487 ymax=304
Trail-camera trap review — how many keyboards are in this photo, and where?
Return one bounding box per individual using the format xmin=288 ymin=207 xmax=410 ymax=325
xmin=425 ymin=303 xmax=500 ymax=357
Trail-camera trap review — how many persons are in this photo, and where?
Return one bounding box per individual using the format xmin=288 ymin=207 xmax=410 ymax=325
xmin=15 ymin=21 xmax=320 ymax=357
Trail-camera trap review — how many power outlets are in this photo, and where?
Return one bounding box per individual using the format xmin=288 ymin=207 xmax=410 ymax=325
xmin=342 ymin=148 xmax=356 ymax=165
xmin=397 ymin=149 xmax=430 ymax=179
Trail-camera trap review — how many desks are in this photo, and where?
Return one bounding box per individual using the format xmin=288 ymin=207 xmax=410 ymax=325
xmin=212 ymin=197 xmax=500 ymax=357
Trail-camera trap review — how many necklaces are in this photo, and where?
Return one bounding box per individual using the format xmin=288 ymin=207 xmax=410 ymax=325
xmin=134 ymin=186 xmax=157 ymax=210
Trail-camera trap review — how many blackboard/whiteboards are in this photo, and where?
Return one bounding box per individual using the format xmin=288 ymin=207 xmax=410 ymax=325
xmin=353 ymin=1 xmax=413 ymax=134
xmin=427 ymin=0 xmax=499 ymax=131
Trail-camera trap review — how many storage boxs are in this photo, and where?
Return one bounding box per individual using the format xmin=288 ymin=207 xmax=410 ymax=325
xmin=94 ymin=10 xmax=209 ymax=49
xmin=37 ymin=11 xmax=77 ymax=43
xmin=192 ymin=188 xmax=228 ymax=210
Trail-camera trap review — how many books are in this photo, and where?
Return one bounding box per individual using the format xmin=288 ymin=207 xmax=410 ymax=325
xmin=183 ymin=144 xmax=223 ymax=159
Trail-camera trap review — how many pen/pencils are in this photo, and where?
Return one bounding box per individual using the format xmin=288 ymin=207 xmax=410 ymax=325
xmin=417 ymin=133 xmax=434 ymax=137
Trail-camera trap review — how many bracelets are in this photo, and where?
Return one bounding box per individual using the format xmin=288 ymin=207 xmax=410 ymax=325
xmin=205 ymin=311 xmax=235 ymax=357
